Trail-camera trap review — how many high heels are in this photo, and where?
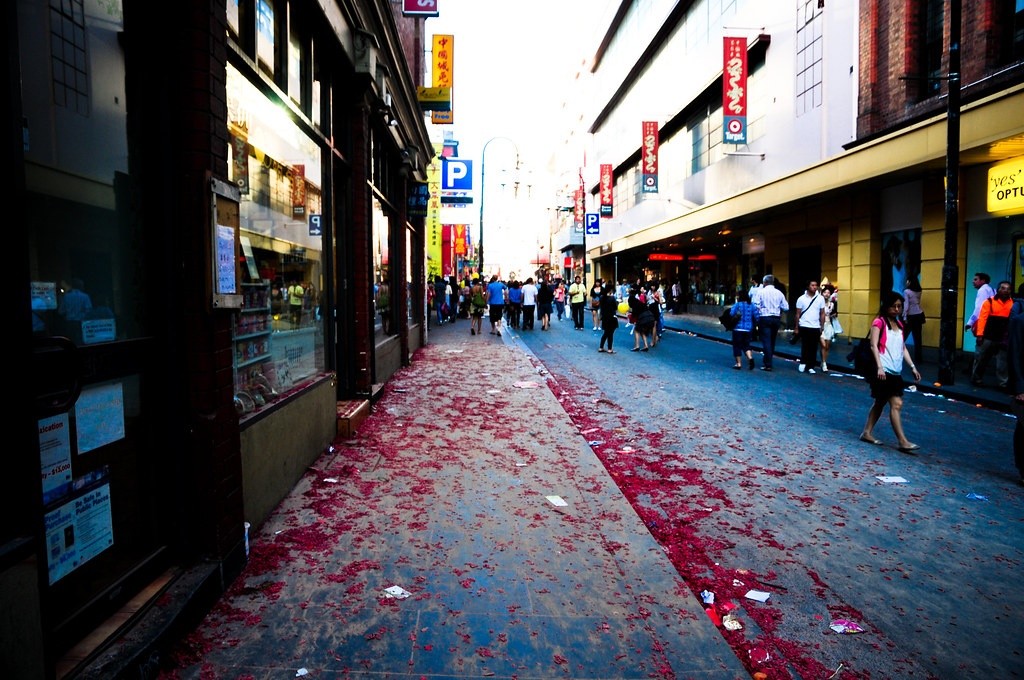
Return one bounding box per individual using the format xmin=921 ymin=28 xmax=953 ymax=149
xmin=630 ymin=347 xmax=641 ymax=352
xmin=640 ymin=348 xmax=649 ymax=352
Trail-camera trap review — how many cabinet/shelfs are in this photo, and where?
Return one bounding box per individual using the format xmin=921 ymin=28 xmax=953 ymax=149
xmin=233 ymin=282 xmax=272 ymax=391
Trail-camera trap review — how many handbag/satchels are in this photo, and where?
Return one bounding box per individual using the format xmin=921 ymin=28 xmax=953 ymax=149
xmin=855 ymin=318 xmax=885 ymax=373
xmin=830 ymin=314 xmax=843 ymax=334
xmin=592 ymin=300 xmax=600 ymax=307
xmin=789 ymin=330 xmax=801 ymax=345
xmin=972 ymin=299 xmax=993 ymax=337
xmin=473 ymin=287 xmax=487 ymax=308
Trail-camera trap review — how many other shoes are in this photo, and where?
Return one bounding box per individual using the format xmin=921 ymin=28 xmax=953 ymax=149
xmin=860 ymin=434 xmax=884 ymax=445
xmin=593 ymin=326 xmax=597 ymax=331
xmin=808 ymin=368 xmax=817 ymax=374
xmin=760 ymin=365 xmax=772 ymax=371
xmin=606 ymin=350 xmax=617 ymax=354
xmin=575 ymin=327 xmax=579 ymax=330
xmin=799 ymin=363 xmax=807 ymax=371
xmin=467 ymin=326 xmax=549 ymax=336
xmin=896 ymin=444 xmax=921 ymax=451
xmin=580 ymin=327 xmax=583 ymax=331
xmin=821 ymin=362 xmax=829 ymax=372
xmin=598 ymin=325 xmax=601 ymax=330
xmin=599 ymin=348 xmax=606 ymax=353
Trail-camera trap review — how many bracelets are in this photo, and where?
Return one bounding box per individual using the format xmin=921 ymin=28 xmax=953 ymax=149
xmin=911 ymin=365 xmax=915 ymax=369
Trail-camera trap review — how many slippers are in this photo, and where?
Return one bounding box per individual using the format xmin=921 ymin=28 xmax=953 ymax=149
xmin=749 ymin=358 xmax=756 ymax=370
xmin=732 ymin=364 xmax=742 ymax=369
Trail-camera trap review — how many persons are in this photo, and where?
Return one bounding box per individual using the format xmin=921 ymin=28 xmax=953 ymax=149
xmin=427 ymin=271 xmax=716 ymax=346
xmin=900 ymin=276 xmax=922 ymax=367
xmin=793 ymin=281 xmax=825 ymax=375
xmin=599 ymin=280 xmax=619 ymax=354
xmin=272 ymin=279 xmax=316 ymax=330
xmin=719 ymin=274 xmax=789 ymax=372
xmin=1008 ymin=319 xmax=1024 ymax=486
xmin=859 ymin=291 xmax=922 ymax=451
xmin=628 ymin=295 xmax=655 ymax=352
xmin=965 ymin=273 xmax=1014 ymax=388
xmin=373 ymin=274 xmax=398 ymax=336
xmin=820 ymin=283 xmax=838 ymax=371
xmin=1007 ymin=283 xmax=1024 ymax=334
